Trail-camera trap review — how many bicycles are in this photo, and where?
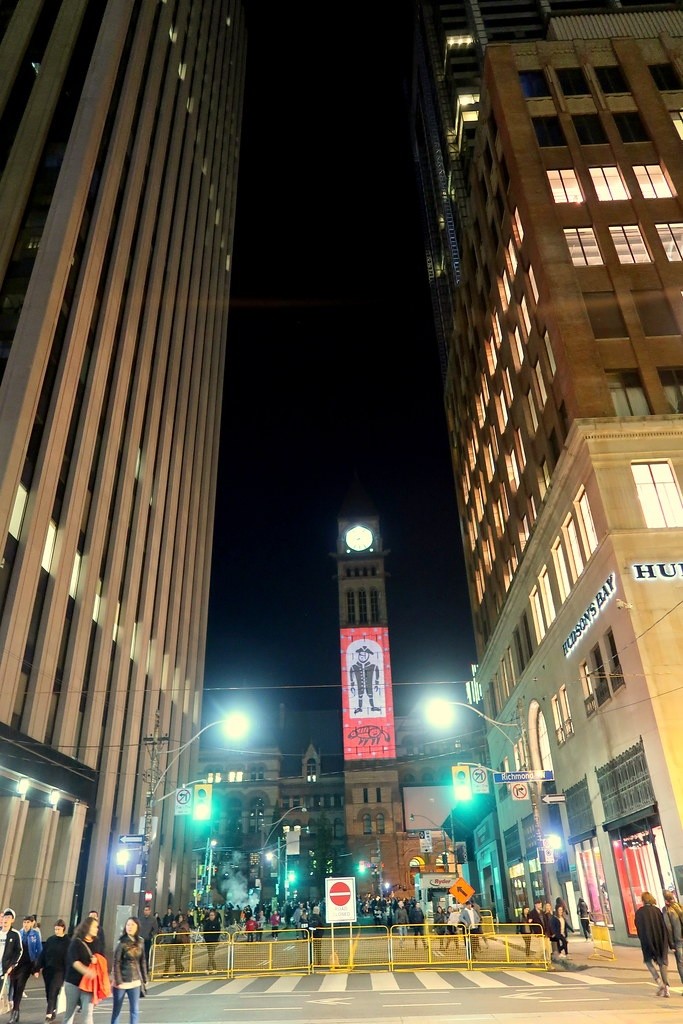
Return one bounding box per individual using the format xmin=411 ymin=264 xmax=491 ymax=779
xmin=188 ymin=919 xmax=256 ymax=949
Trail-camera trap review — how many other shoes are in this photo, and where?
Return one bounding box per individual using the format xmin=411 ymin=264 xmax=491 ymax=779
xmin=566 ymin=954 xmax=573 ymax=960
xmin=211 ymin=970 xmax=217 ymax=975
xmin=44 ymin=1013 xmax=56 ymax=1024
xmin=21 ymin=992 xmax=27 ymax=998
xmin=558 ymin=954 xmax=566 ymax=958
xmin=204 ymin=970 xmax=209 ymax=975
xmin=657 ymin=984 xmax=665 ymax=997
xmin=664 ymin=985 xmax=671 ymax=997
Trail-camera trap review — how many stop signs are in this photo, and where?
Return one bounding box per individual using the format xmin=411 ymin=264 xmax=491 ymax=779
xmin=330 ymin=882 xmax=351 ymax=906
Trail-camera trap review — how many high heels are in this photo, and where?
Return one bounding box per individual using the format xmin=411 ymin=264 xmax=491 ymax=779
xmin=8 ymin=1010 xmax=19 ymax=1024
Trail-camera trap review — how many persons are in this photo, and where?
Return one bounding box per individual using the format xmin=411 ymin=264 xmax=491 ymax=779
xmin=516 ymin=897 xmax=575 ymax=965
xmin=356 ymin=893 xmax=489 ymax=961
xmin=0 ymin=908 xmax=107 ymax=1024
xmin=577 ymin=898 xmax=593 ymax=941
xmin=111 ymin=915 xmax=148 ymax=1024
xmin=634 ymin=892 xmax=671 ymax=997
xmin=662 ymin=890 xmax=683 ymax=996
xmin=140 ymin=897 xmax=326 ymax=978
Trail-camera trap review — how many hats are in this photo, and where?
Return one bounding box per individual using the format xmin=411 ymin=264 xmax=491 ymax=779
xmin=2 ymin=908 xmax=16 ymax=919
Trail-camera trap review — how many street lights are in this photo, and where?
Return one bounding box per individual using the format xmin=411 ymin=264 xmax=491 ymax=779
xmin=278 ymin=826 xmax=310 ymax=884
xmin=409 ymin=809 xmax=459 ymax=878
xmin=428 ymin=701 xmax=562 ymax=963
xmin=137 ymin=717 xmax=245 ymax=920
xmin=259 ymin=803 xmax=307 ymax=911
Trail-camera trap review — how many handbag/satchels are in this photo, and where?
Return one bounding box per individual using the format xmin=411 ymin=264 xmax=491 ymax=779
xmin=55 ymin=981 xmax=67 ymax=1014
xmin=139 ymin=979 xmax=145 ymax=998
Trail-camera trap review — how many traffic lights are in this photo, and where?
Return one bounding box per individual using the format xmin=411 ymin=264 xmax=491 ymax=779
xmin=452 ymin=766 xmax=471 ymax=799
xmin=359 ymin=861 xmax=365 ymax=872
xmin=288 ymin=871 xmax=294 ymax=882
xmin=206 ymin=885 xmax=211 ymax=893
xmin=193 ymin=785 xmax=212 ymax=820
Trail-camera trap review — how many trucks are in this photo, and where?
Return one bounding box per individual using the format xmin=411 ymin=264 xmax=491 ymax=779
xmin=413 ymin=873 xmax=458 ymax=932
